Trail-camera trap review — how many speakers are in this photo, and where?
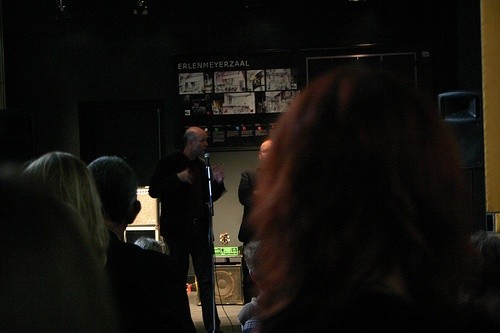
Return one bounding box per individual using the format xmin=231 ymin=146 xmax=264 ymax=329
xmin=195 ymin=264 xmax=245 ymax=306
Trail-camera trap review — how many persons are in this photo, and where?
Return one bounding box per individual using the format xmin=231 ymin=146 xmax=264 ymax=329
xmin=237 ymin=136 xmax=275 ymax=303
xmin=84 ymin=156 xmax=199 ymax=333
xmin=149 ymin=126 xmax=223 ymax=333
xmin=25 ymin=151 xmax=110 ymax=268
xmin=0 ymin=170 xmax=119 ymax=333
xmin=245 ymin=62 xmax=490 ymax=333
xmin=237 ymin=239 xmax=280 ymax=326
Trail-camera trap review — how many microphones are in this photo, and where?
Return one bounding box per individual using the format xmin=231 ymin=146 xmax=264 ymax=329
xmin=204 ymin=153 xmax=210 ymax=168
